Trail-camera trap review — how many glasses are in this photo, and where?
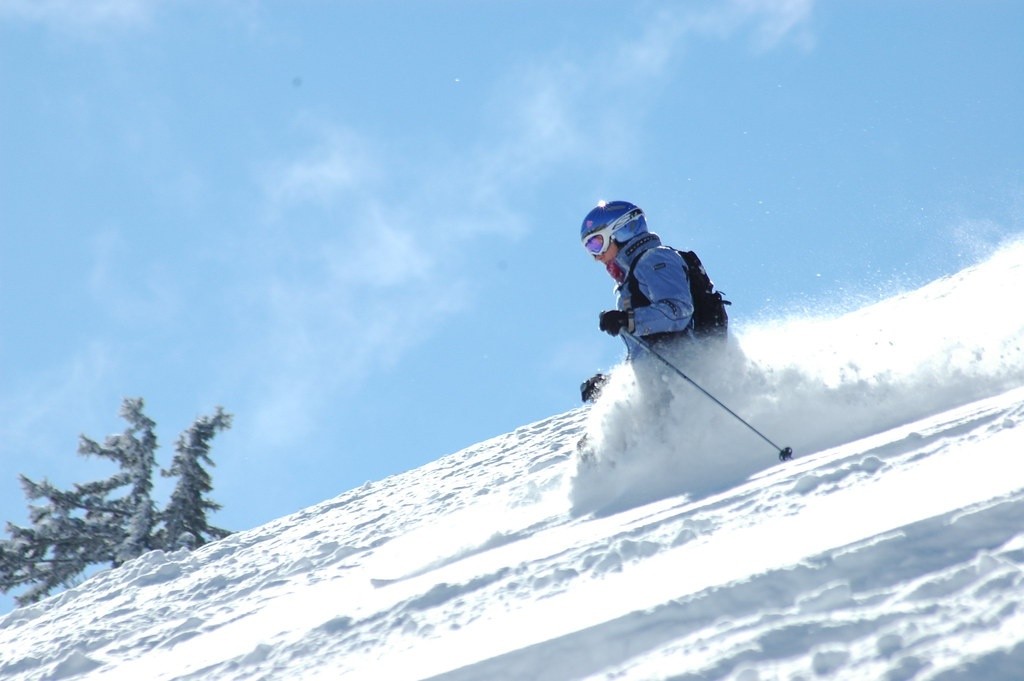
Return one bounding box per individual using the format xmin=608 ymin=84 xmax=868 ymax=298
xmin=583 ymin=207 xmax=644 ymax=259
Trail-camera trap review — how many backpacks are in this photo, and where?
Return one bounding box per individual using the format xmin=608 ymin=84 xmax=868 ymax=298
xmin=627 ymin=241 xmax=733 ymax=350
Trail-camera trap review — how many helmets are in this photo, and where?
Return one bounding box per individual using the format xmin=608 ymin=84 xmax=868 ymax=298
xmin=581 ymin=200 xmax=648 ymax=250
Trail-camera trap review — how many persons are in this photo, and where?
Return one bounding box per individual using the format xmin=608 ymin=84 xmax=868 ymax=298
xmin=580 ymin=201 xmax=698 ymax=402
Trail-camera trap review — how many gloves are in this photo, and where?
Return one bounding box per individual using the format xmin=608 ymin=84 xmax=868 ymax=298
xmin=598 ymin=309 xmax=628 ymax=337
xmin=581 ymin=374 xmax=605 ymax=403
